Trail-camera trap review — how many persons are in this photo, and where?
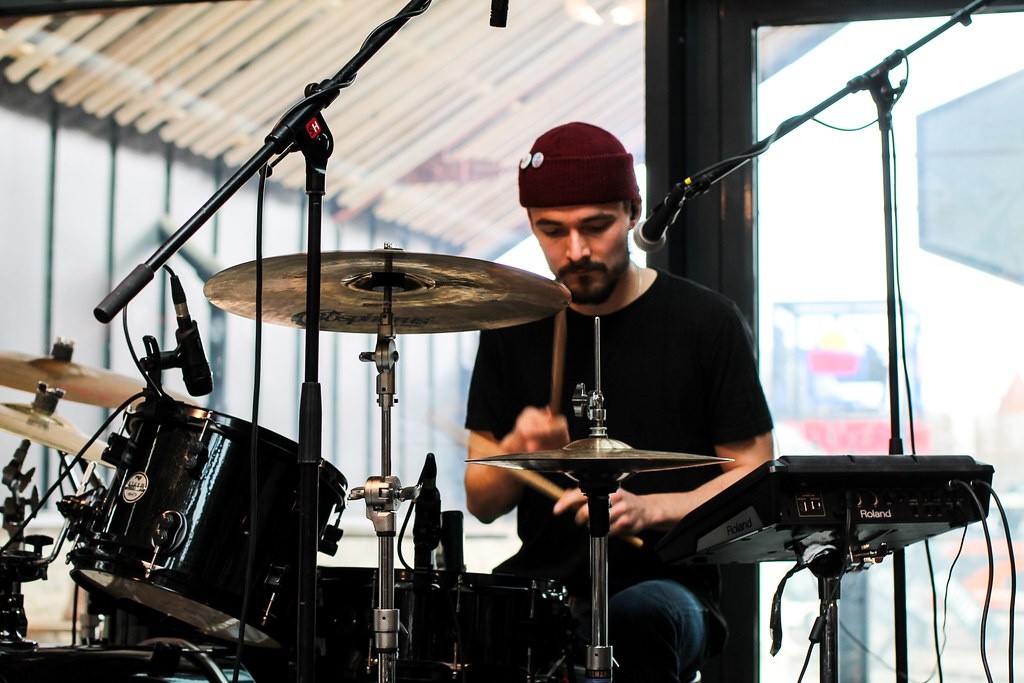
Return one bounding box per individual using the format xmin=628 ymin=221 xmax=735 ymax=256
xmin=460 ymin=122 xmax=779 ymax=683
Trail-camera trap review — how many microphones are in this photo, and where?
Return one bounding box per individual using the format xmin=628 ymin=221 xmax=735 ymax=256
xmin=170 ymin=272 xmax=212 ymax=397
xmin=412 ymin=453 xmax=442 ymax=570
xmin=489 ymin=0 xmax=508 ymax=28
xmin=632 ymin=182 xmax=685 ymax=254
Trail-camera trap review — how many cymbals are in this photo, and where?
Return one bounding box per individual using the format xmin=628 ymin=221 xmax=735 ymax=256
xmin=464 ymin=439 xmax=734 ymax=482
xmin=0 ymin=405 xmax=116 ymax=470
xmin=203 ymin=251 xmax=571 ymax=335
xmin=0 ymin=350 xmax=198 ymax=409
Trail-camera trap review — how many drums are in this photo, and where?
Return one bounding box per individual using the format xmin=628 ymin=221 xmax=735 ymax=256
xmin=317 ymin=565 xmax=572 ymax=683
xmin=69 ymin=401 xmax=348 ymax=645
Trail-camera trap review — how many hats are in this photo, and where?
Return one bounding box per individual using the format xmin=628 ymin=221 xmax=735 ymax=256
xmin=518 ymin=122 xmax=639 ymax=208
